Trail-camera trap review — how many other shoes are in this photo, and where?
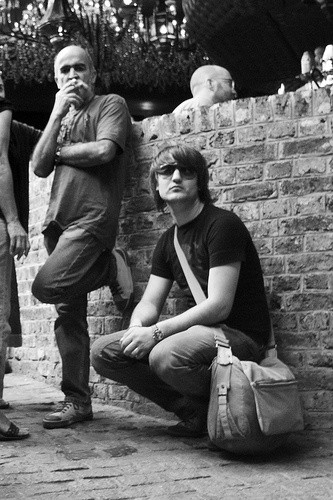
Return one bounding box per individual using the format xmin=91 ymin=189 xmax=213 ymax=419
xmin=0 ymin=400 xmax=9 ymax=409
xmin=168 ymin=416 xmax=207 ymax=436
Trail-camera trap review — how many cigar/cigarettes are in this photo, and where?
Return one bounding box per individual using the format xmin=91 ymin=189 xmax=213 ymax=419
xmin=72 ymin=82 xmax=76 ymax=86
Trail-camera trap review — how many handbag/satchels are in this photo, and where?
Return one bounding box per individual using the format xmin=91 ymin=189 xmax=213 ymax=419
xmin=208 ymin=357 xmax=303 ymax=458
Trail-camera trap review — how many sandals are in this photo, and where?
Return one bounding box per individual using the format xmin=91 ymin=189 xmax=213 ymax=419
xmin=0 ymin=422 xmax=30 ymax=440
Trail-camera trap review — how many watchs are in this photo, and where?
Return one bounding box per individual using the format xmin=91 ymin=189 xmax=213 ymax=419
xmin=151 ymin=325 xmax=165 ymax=342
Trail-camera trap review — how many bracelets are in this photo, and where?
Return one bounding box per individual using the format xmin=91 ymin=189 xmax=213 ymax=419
xmin=55 ymin=144 xmax=64 ymax=165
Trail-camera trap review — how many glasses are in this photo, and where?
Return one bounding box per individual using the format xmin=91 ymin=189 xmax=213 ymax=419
xmin=155 ymin=165 xmax=198 ymax=179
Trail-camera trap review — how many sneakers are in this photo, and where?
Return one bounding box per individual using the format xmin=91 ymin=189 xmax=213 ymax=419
xmin=43 ymin=401 xmax=93 ymax=428
xmin=111 ymin=247 xmax=133 ymax=312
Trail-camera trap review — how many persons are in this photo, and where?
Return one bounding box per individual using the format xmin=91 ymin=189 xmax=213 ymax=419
xmin=90 ymin=144 xmax=270 ymax=450
xmin=0 ymin=68 xmax=44 ymax=441
xmin=32 ymin=46 xmax=134 ymax=429
xmin=172 ymin=64 xmax=237 ymax=112
xmin=278 ymin=45 xmax=333 ymax=95
